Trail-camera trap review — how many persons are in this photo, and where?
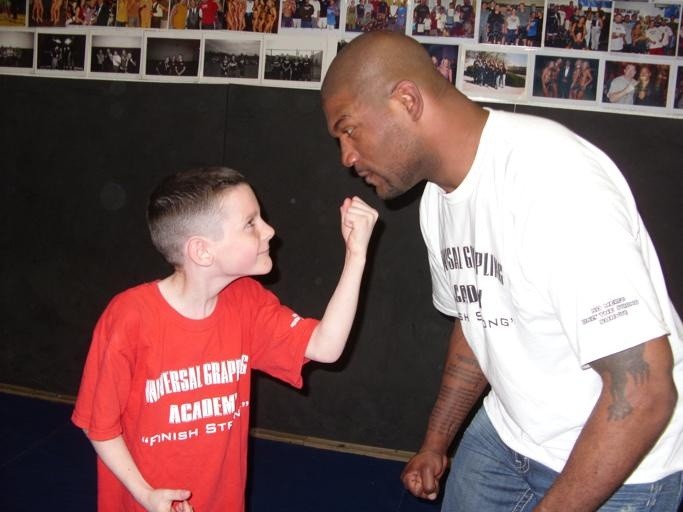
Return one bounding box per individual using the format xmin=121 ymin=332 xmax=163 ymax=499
xmin=32 ymin=0 xmax=673 ymax=105
xmin=321 ymin=30 xmax=683 ymax=512
xmin=70 ymin=166 xmax=379 ymax=512
